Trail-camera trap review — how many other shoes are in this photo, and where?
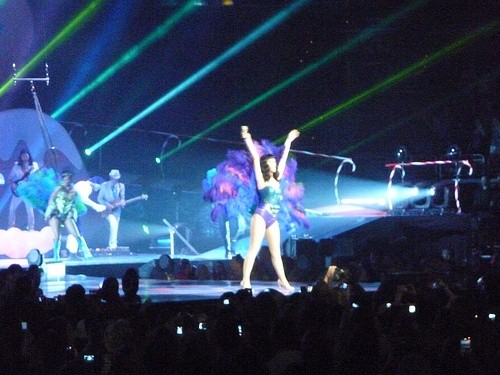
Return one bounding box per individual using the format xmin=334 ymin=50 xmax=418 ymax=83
xmin=225 ymin=251 xmax=238 ymax=259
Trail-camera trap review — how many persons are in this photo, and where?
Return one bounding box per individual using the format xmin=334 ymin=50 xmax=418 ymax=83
xmin=97 ymin=169 xmax=126 ymax=251
xmin=218 ymin=218 xmax=239 ymax=260
xmin=0 ymin=247 xmax=500 ymax=375
xmin=66 ymin=175 xmax=106 ymax=261
xmin=241 ymin=126 xmax=300 ymax=291
xmin=44 ymin=169 xmax=92 ymax=261
xmin=474 ymin=176 xmax=495 ymax=210
xmin=8 ymin=149 xmax=39 ymax=230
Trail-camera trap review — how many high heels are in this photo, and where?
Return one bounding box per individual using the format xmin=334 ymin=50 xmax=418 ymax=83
xmin=278 ymin=279 xmax=294 ymax=292
xmin=240 ymin=280 xmax=254 ymax=293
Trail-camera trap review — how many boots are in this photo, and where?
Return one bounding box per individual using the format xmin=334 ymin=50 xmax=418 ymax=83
xmin=77 ymin=236 xmax=94 ymax=259
xmin=53 ymin=241 xmax=62 ymax=262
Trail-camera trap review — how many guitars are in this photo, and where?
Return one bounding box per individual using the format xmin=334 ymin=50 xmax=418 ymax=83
xmin=99 ymin=193 xmax=149 ymax=215
xmin=10 ymin=166 xmax=48 ymax=197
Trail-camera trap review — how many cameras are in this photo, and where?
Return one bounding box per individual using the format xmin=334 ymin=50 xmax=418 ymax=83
xmin=332 ymin=268 xmax=345 ymax=282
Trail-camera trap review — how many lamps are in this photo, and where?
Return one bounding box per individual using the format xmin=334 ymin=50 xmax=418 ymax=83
xmin=28 ymin=249 xmax=43 ymax=267
xmin=156 ymin=255 xmax=177 ymax=280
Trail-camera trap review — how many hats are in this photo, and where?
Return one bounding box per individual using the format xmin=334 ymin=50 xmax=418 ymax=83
xmin=109 ymin=169 xmax=121 ymax=180
